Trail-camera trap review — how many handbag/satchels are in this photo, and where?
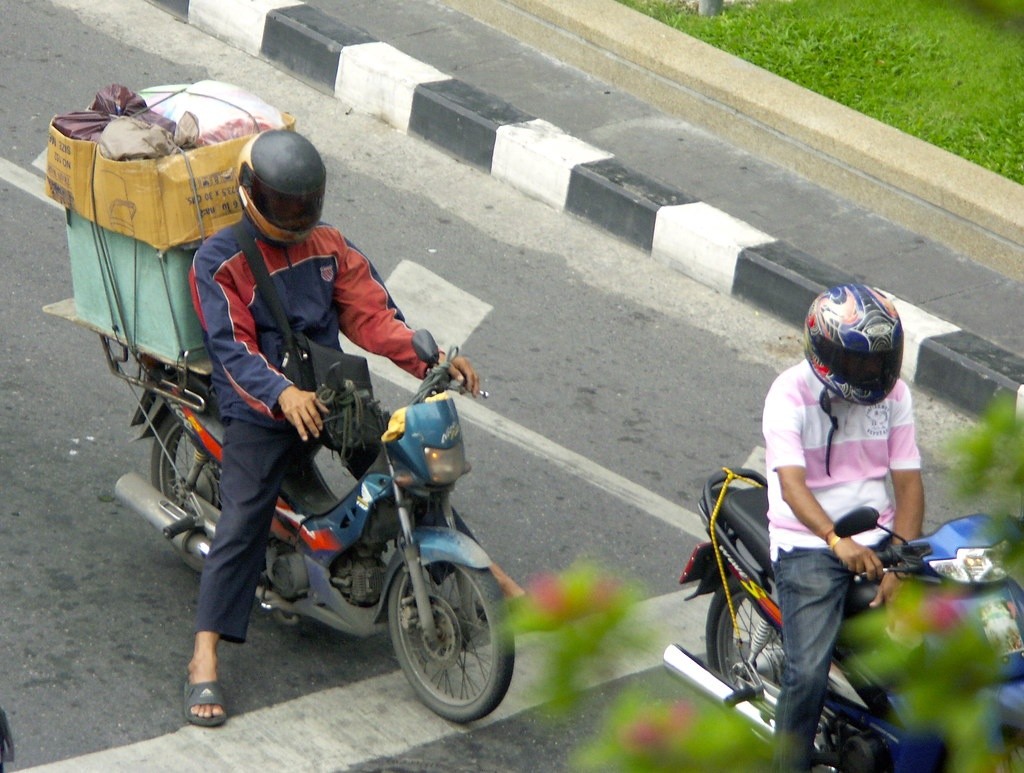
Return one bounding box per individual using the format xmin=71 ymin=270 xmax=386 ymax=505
xmin=280 ymin=333 xmax=383 ymax=447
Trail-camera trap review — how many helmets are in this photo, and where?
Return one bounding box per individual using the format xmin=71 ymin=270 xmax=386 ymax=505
xmin=235 ymin=130 xmax=325 ymax=247
xmin=806 ymin=284 xmax=904 ymax=404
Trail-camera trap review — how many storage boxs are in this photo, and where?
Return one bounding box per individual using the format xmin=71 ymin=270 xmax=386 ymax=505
xmin=63 ymin=207 xmax=210 ymax=364
xmin=46 ymin=111 xmax=297 ymax=250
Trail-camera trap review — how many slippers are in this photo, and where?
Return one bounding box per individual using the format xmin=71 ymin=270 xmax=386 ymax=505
xmin=184 ymin=678 xmax=225 ymax=726
xmin=477 ymin=592 xmax=558 ymax=631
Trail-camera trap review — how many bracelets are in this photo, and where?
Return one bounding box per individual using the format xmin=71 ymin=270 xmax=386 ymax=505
xmin=830 ymin=536 xmax=841 ymax=551
xmin=824 ymin=529 xmax=833 ymax=545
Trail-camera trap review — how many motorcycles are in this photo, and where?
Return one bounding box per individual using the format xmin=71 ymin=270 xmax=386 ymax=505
xmin=663 ymin=469 xmax=1023 ymax=773
xmin=99 ymin=331 xmax=518 ymax=725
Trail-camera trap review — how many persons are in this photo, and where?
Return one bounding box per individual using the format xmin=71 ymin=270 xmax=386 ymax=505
xmin=181 ymin=129 xmax=480 ymax=728
xmin=761 ymin=281 xmax=925 ymax=773
